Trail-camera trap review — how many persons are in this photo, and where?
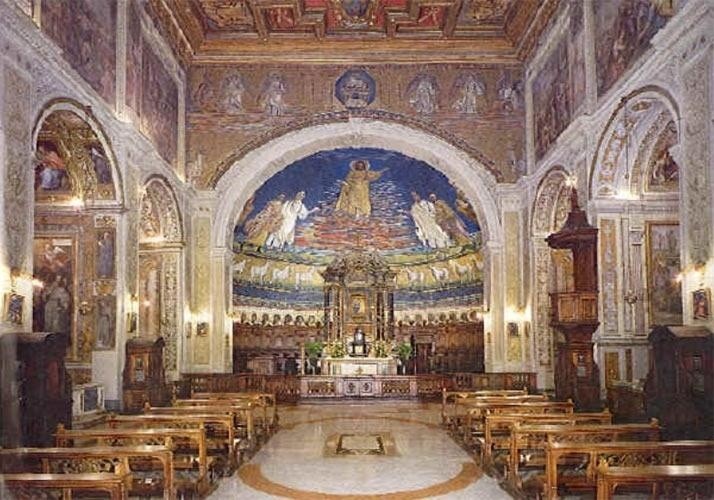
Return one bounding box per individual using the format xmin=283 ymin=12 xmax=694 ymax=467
xmin=334 ymin=160 xmax=390 ymax=217
xmin=38 ymin=143 xmax=65 ymax=190
xmin=246 ymin=191 xmax=321 ymax=251
xmin=410 ymin=190 xmax=473 ymax=249
xmin=33 ymin=242 xmax=73 ymax=333
xmin=98 ymin=232 xmax=114 ymax=278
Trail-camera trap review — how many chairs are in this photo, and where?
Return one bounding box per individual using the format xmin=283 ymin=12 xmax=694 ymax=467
xmin=440 ymin=384 xmax=713 ymax=500
xmin=1 ymin=390 xmax=278 ymax=499
